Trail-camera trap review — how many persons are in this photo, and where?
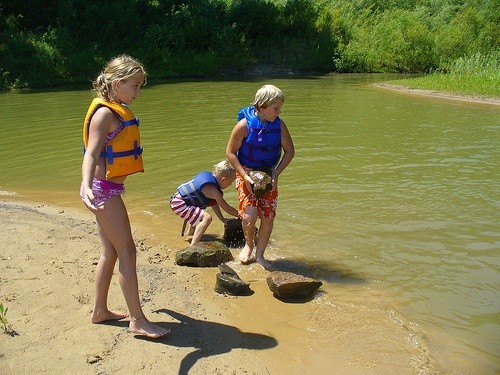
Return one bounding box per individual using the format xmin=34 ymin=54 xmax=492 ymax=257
xmin=80 ymin=56 xmax=171 ymax=338
xmin=226 ymin=85 xmax=295 ymax=268
xmin=170 ymin=160 xmax=239 ymax=246
